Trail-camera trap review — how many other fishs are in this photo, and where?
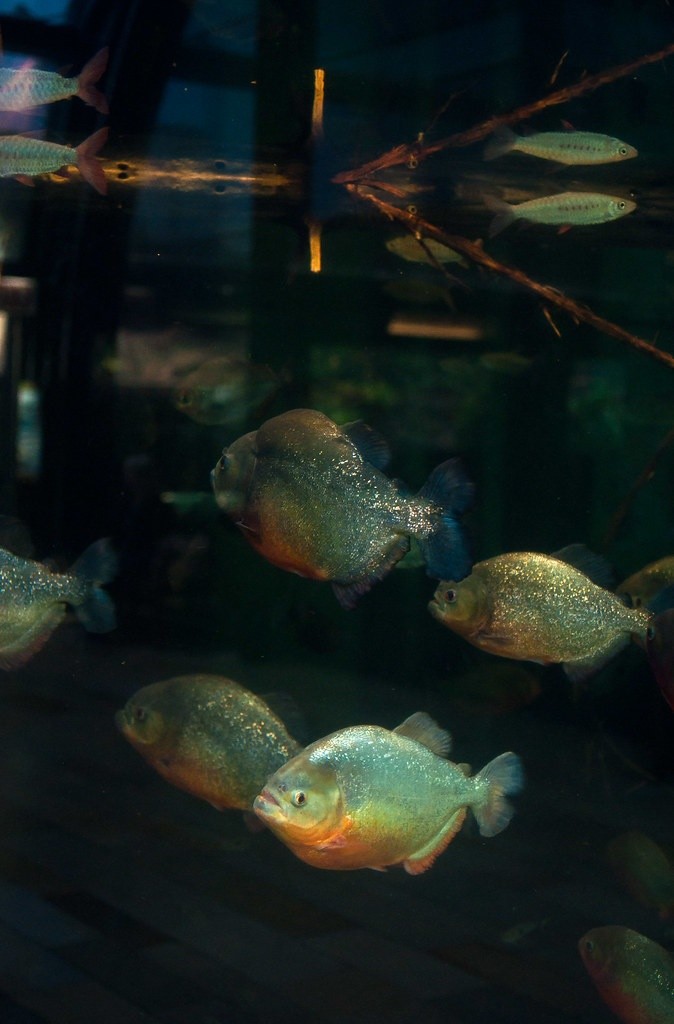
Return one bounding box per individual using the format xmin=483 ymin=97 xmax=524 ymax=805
xmin=0 ymin=46 xmax=674 ymax=1024
